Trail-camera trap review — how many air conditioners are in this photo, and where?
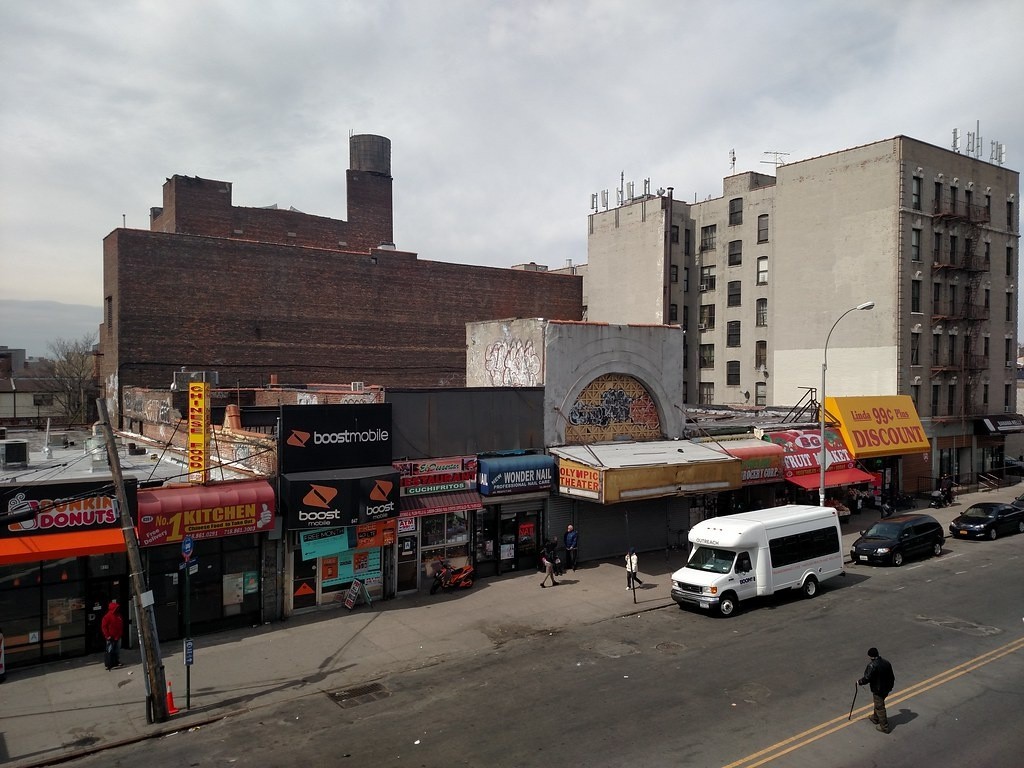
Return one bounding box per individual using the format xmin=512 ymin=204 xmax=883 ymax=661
xmin=351 ymin=382 xmax=364 ymax=391
xmin=699 ymin=284 xmax=708 ymax=291
xmin=698 ymin=323 xmax=707 ymax=329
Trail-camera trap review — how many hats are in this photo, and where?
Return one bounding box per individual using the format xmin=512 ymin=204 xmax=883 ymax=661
xmin=868 ymin=648 xmax=879 ymax=657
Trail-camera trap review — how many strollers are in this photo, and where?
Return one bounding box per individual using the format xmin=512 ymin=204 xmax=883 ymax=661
xmin=928 ymin=490 xmax=945 ymax=508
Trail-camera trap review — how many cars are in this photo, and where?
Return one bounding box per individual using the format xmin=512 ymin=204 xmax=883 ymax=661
xmin=1011 ymin=493 xmax=1024 ymax=507
xmin=850 ymin=513 xmax=946 ymax=566
xmin=948 ymin=502 xmax=1024 ymax=541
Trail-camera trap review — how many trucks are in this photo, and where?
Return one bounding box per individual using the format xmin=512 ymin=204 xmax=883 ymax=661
xmin=671 ymin=504 xmax=845 ymax=617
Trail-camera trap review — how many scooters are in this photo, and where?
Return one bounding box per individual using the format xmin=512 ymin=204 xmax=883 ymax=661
xmin=429 ymin=558 xmax=475 ymax=594
xmin=877 ymin=491 xmax=920 ymax=517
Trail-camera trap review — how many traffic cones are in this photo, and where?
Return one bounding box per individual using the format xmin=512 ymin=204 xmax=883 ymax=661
xmin=167 ymin=681 xmax=180 ymax=714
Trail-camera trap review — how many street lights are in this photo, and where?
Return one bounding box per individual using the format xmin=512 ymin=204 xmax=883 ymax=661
xmin=819 ymin=301 xmax=875 ymax=507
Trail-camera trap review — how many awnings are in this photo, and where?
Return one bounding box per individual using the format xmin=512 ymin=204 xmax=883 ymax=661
xmin=478 ymin=454 xmax=554 ymax=495
xmin=704 ymin=439 xmax=785 ymax=486
xmin=0 ymin=527 xmax=128 ymax=565
xmin=982 ymin=414 xmax=1024 ymax=436
xmin=135 ymin=480 xmax=275 ymax=547
xmin=281 ymin=465 xmax=400 ymax=530
xmin=786 ymin=468 xmax=876 ymax=491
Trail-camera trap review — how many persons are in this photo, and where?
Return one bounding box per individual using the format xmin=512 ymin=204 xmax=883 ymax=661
xmin=882 ymin=500 xmax=892 ymax=517
xmin=102 ymin=602 xmax=124 ymax=669
xmin=941 ymin=473 xmax=953 ymax=507
xmin=562 ymin=525 xmax=578 ymax=570
xmin=625 ymin=552 xmax=644 ymax=590
xmin=857 ymin=647 xmax=895 ymax=734
xmin=538 ymin=536 xmax=559 ymax=587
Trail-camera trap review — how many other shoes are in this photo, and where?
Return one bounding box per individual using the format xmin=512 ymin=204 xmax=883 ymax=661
xmin=553 ymin=582 xmax=559 ymax=586
xmin=869 ymin=715 xmax=879 ymax=724
xmin=639 ymin=581 xmax=644 ymax=587
xmin=876 ymin=725 xmax=884 ymax=732
xmin=540 ymin=583 xmax=546 ymax=588
xmin=626 ymin=586 xmax=631 ymax=590
